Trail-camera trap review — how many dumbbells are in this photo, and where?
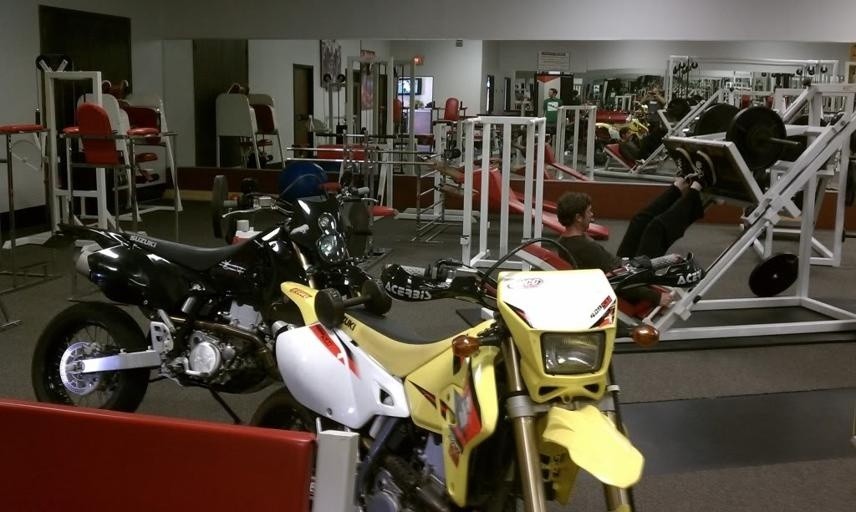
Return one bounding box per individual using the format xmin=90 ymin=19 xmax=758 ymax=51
xmin=315 ymin=278 xmax=391 ymax=328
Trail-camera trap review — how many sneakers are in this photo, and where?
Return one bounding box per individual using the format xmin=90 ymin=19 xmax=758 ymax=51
xmin=695 ymin=151 xmax=717 ymax=187
xmin=675 ymin=148 xmax=696 ymax=184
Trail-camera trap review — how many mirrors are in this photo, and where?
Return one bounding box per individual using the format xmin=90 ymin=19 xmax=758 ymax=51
xmin=161 ymin=38 xmax=856 ymax=193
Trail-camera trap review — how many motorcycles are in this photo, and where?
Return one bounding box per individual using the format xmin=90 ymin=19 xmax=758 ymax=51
xmin=246 ymin=235 xmax=658 ymax=511
xmin=30 ymin=148 xmax=391 ymax=416
xmin=531 ymin=96 xmax=651 ymax=167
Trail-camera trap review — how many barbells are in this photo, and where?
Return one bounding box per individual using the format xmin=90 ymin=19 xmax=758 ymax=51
xmin=211 ymin=177 xmax=373 ymax=257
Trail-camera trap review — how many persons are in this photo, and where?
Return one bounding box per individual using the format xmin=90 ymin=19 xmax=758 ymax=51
xmin=557 ymin=147 xmax=717 ymax=307
xmin=618 ymin=120 xmax=668 ymax=161
xmin=640 ymin=88 xmax=666 ymax=127
xmin=566 ymin=91 xmax=582 ymax=105
xmin=543 ymin=88 xmax=564 ymax=123
